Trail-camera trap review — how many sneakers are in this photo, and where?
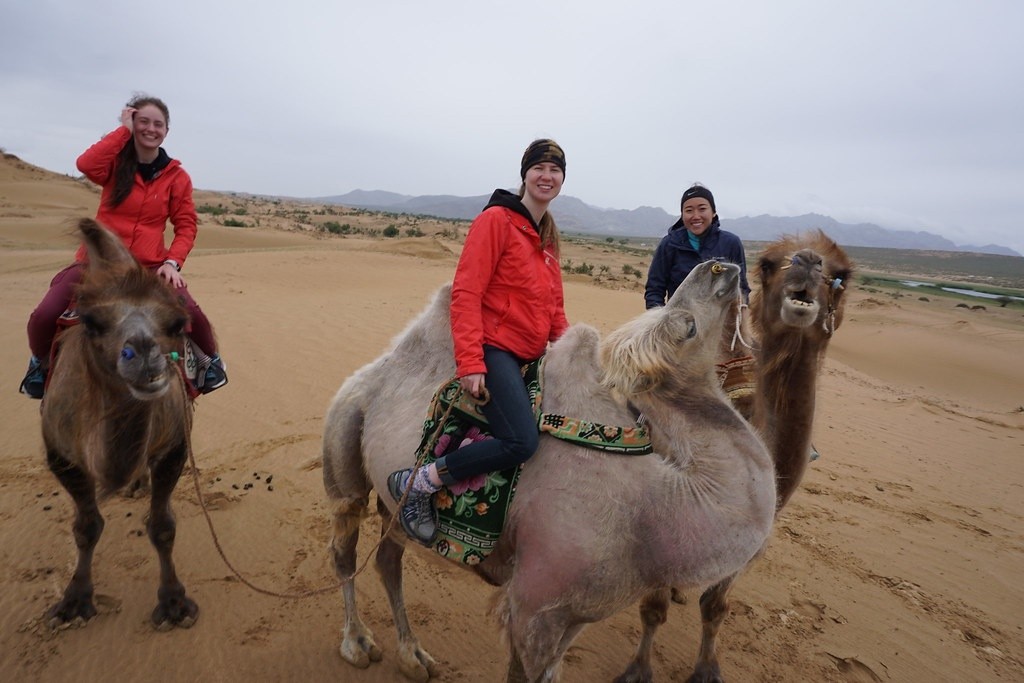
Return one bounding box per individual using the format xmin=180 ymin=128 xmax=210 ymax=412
xmin=22 ymin=356 xmax=48 ymax=398
xmin=203 ymin=356 xmax=226 ymax=388
xmin=387 ymin=468 xmax=438 ymax=547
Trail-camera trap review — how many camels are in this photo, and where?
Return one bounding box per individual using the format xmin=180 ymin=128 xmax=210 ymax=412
xmin=614 ymin=225 xmax=856 ymax=683
xmin=38 ymin=216 xmax=200 ymax=631
xmin=294 ymin=256 xmax=777 ymax=683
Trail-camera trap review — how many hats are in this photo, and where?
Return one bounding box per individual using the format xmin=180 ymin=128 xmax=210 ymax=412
xmin=521 ymin=139 xmax=566 ymax=186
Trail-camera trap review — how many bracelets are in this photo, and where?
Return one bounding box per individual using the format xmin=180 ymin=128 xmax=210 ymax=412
xmin=164 ymin=260 xmax=180 ymax=269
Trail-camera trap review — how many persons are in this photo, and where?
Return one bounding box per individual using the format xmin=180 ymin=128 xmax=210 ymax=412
xmin=644 ymin=185 xmax=751 ymax=311
xmin=388 ymin=138 xmax=571 ymax=538
xmin=25 ymin=97 xmax=228 ymax=399
